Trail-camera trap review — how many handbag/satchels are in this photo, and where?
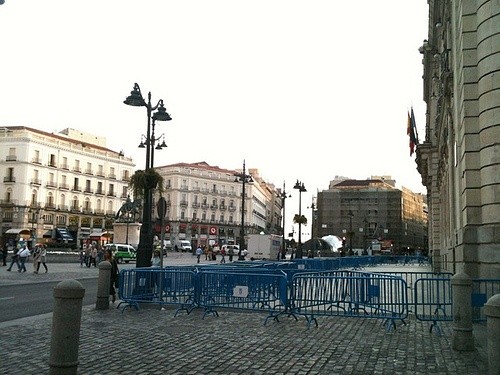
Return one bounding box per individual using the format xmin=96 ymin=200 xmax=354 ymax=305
xmin=114 ymin=273 xmax=120 ymax=287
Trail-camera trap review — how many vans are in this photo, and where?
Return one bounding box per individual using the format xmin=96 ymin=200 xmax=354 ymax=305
xmin=102 ymin=244 xmax=137 ymax=264
xmin=221 ymin=245 xmax=247 ymax=255
xmin=174 ymin=240 xmax=192 ymax=252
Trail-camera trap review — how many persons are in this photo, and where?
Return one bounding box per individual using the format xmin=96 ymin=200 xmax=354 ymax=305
xmin=0 ymin=240 xmax=428 ymax=274
xmin=150 ymin=250 xmax=163 ymax=292
xmin=102 ymin=250 xmax=120 ymax=303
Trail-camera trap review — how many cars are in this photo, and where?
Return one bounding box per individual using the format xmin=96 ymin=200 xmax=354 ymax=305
xmin=153 ymin=243 xmax=167 ymax=258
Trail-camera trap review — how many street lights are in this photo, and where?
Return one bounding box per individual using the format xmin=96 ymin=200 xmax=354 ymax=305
xmin=121 ymin=83 xmax=172 ymax=299
xmin=276 ymin=182 xmax=291 ymax=258
xmin=293 ymin=179 xmax=307 ymax=258
xmin=28 ymin=194 xmax=41 ymax=228
xmin=346 ymin=210 xmax=355 ymax=255
xmin=101 ymin=215 xmax=113 ymax=244
xmin=234 ymin=158 xmax=254 ymax=260
xmin=363 ymin=218 xmax=369 ymax=255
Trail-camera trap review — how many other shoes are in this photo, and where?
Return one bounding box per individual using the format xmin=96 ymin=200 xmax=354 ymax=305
xmin=34 ymin=271 xmax=38 ymax=273
xmin=45 ymin=269 xmax=48 ymax=274
xmin=23 ymin=270 xmax=26 ymax=272
xmin=6 ymin=268 xmax=11 ymax=271
xmin=112 ymin=294 xmax=115 ymax=303
xmin=19 ymin=265 xmax=22 ymax=270
xmin=18 ymin=270 xmax=21 ymax=273
